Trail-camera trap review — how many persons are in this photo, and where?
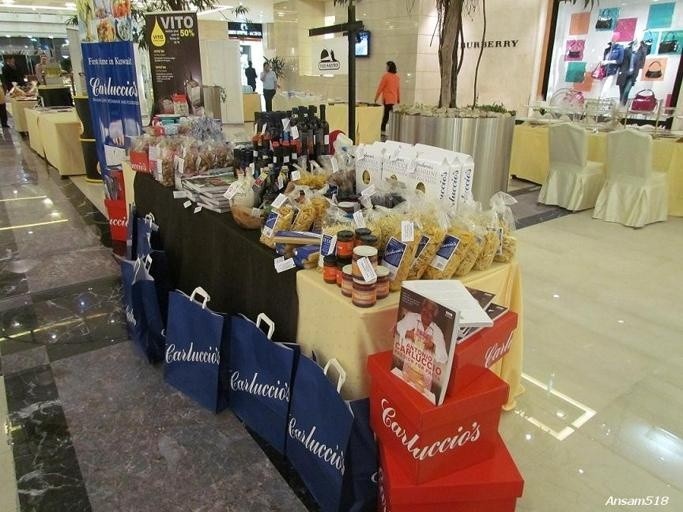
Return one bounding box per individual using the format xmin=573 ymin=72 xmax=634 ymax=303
xmin=393 ymin=299 xmax=448 ymax=392
xmin=32 ymin=54 xmax=52 ymax=86
xmin=1 ymin=55 xmax=27 ymax=89
xmin=245 ymin=61 xmax=258 ymax=92
xmin=258 ymin=63 xmax=278 ymax=112
xmin=611 ymin=31 xmax=647 ymax=106
xmin=373 ymin=61 xmax=401 ymax=133
xmin=593 ymin=30 xmax=625 ymax=98
xmin=0 ymin=56 xmax=10 ymax=129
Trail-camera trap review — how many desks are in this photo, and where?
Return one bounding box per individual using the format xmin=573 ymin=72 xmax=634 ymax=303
xmin=122 ymin=167 xmax=527 ymax=411
xmin=5 ymin=96 xmax=39 ymax=141
xmin=22 ymin=107 xmax=87 ymax=180
xmin=307 ymin=99 xmax=385 ymax=146
xmin=243 ymin=93 xmax=263 ymax=122
xmin=510 ymin=119 xmax=683 ymax=219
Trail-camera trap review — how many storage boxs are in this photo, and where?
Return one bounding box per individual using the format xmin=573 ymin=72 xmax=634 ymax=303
xmin=104 ymin=199 xmax=126 ymax=242
xmin=105 ymin=170 xmax=126 ymax=202
xmin=366 ymin=308 xmax=526 ymax=512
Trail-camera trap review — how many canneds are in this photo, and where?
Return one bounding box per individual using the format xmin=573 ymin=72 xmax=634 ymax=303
xmin=323 ymin=228 xmax=389 ymax=308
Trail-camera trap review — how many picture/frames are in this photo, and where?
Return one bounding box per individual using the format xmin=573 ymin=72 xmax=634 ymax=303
xmin=355 ymin=31 xmax=370 ymax=57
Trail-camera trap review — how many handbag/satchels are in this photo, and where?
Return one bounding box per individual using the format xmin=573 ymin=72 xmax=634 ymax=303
xmin=658 ymin=31 xmax=680 ymax=53
xmin=119 ymin=203 xmax=377 ymax=512
xmin=355 ymin=140 xmax=474 ymax=212
xmin=645 ymin=61 xmax=662 ymax=78
xmin=591 ymin=62 xmax=603 ymax=80
xmin=631 ymin=89 xmax=656 ymax=112
xmin=568 ymin=50 xmax=580 ymax=58
xmin=595 ymin=8 xmax=612 ymax=29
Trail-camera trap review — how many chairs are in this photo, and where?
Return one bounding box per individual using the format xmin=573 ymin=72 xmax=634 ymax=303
xmin=591 ymin=128 xmax=670 ymax=230
xmin=536 ymin=122 xmax=605 ymax=213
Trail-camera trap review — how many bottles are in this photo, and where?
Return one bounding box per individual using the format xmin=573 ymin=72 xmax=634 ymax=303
xmin=230 ymin=103 xmax=331 ymax=208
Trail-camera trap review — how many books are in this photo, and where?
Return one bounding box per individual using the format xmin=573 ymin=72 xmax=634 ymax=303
xmin=386 ymin=285 xmax=462 ymax=407
xmin=179 ymin=171 xmax=242 ymax=217
xmin=452 ymin=304 xmax=508 ymax=344
xmin=403 ymin=279 xmax=495 ymax=330
xmin=449 ymin=286 xmax=494 ymax=329
xmin=453 ymin=321 xmax=471 ymax=337
